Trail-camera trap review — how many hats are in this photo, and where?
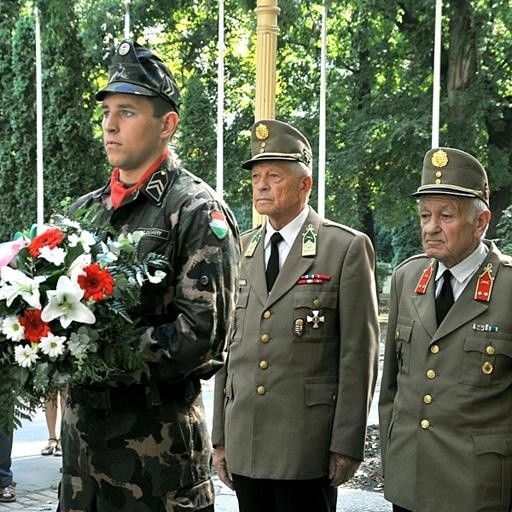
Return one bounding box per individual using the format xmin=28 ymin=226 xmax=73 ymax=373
xmin=238 ymin=119 xmax=313 ymax=170
xmin=94 ymin=38 xmax=181 ymax=116
xmin=408 ymin=146 xmax=492 ymax=207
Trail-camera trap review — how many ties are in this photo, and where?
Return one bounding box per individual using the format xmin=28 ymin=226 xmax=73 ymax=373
xmin=265 ymin=230 xmax=284 ymax=292
xmin=434 ymin=270 xmax=455 ymax=325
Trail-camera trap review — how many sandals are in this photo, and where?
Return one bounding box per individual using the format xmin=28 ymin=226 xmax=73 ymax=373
xmin=0 ymin=477 xmax=16 ymax=503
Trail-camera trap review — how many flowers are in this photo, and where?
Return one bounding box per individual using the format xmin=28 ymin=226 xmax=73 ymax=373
xmin=0 ymin=213 xmax=169 ymax=439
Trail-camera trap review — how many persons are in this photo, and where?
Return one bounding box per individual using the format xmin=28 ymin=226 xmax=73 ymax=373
xmin=0 ymin=389 xmax=17 ymax=499
xmin=55 ymin=39 xmax=243 ymax=512
xmin=212 ymin=120 xmax=381 ymax=511
xmin=40 ymin=385 xmax=69 ymax=455
xmin=377 ymin=147 xmax=510 ymax=512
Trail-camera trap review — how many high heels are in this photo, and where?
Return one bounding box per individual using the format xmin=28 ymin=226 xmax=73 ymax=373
xmin=54 ymin=437 xmax=65 ymax=457
xmin=41 ymin=437 xmax=54 ymax=456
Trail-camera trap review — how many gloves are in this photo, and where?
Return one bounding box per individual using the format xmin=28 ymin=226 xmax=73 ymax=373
xmin=213 ymin=445 xmax=236 ymax=493
xmin=329 ymin=456 xmax=362 ymax=488
